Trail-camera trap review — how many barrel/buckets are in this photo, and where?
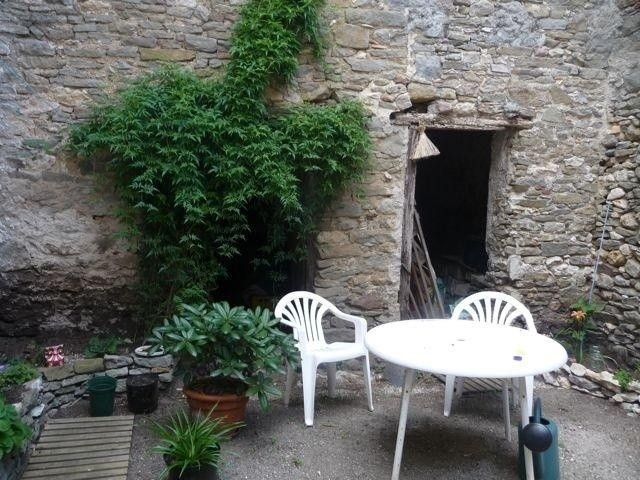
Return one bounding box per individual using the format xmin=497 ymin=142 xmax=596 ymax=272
xmin=125 ymin=359 xmax=158 ymax=414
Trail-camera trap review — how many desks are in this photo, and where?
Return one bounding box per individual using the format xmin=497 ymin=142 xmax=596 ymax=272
xmin=364 ymin=319 xmax=568 ymax=480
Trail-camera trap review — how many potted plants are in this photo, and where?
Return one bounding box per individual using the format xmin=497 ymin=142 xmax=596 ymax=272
xmin=147 ymin=401 xmax=247 ymax=480
xmin=141 ymin=303 xmax=303 ymax=437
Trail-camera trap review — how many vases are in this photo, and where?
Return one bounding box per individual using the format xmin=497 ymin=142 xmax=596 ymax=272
xmin=126 ymin=372 xmax=158 ymax=414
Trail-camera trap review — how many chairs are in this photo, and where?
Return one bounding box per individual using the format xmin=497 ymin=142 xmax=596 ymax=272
xmin=275 ymin=291 xmax=373 ymax=426
xmin=443 ymin=291 xmax=537 ymax=417
